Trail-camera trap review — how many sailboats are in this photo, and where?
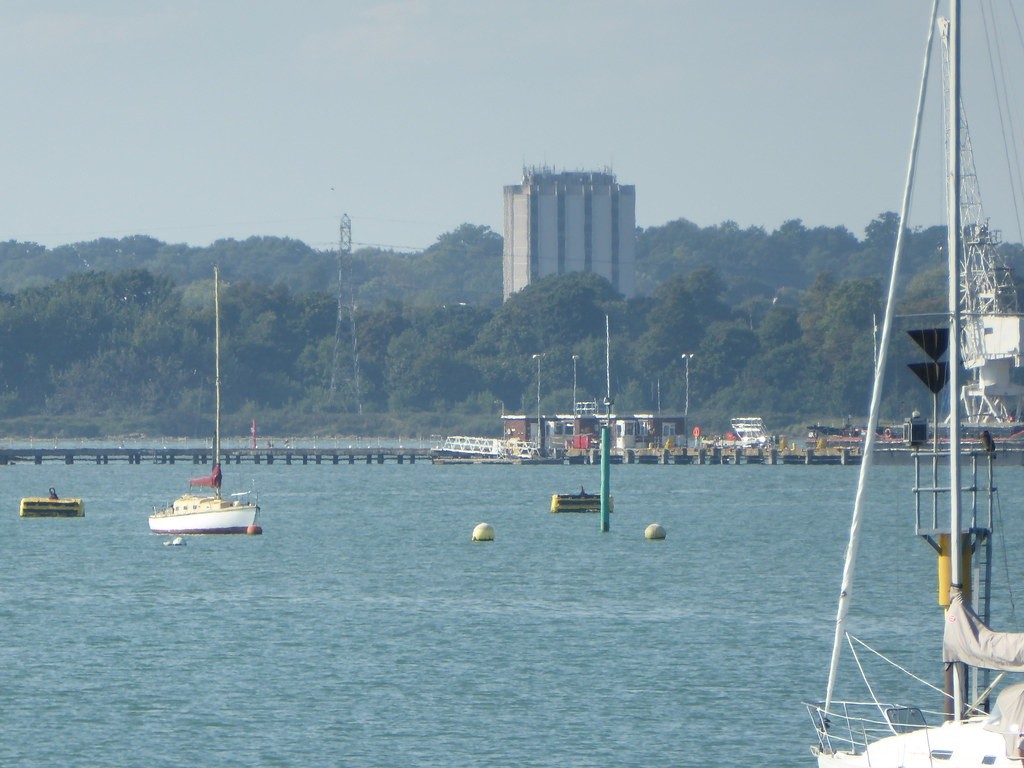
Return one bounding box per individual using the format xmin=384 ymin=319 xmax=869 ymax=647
xmin=148 ymin=264 xmax=261 ymax=535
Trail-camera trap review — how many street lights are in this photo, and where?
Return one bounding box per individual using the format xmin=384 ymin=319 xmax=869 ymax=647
xmin=532 ymin=352 xmax=546 ymax=448
xmin=682 ymin=353 xmax=696 ymax=448
xmin=573 ymin=355 xmax=580 ymax=419
